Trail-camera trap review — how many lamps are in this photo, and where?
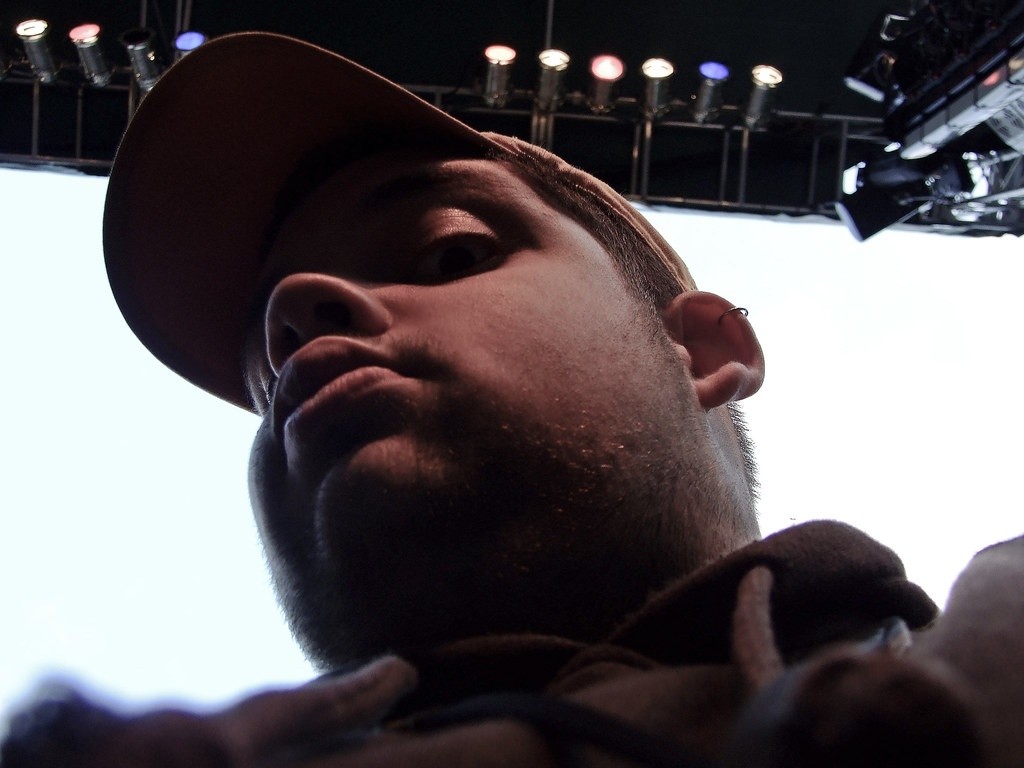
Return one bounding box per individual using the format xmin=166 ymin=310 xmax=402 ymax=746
xmin=120 ymin=27 xmax=164 ymax=93
xmin=833 ymin=150 xmax=966 ymax=243
xmin=11 ymin=16 xmax=65 ymax=85
xmin=743 ymin=65 xmax=784 ymax=127
xmin=69 ymin=23 xmax=119 ymax=89
xmin=172 ymin=30 xmax=209 ymax=59
xmin=843 ymin=41 xmax=910 ymax=104
xmin=534 ymin=49 xmax=570 ymax=112
xmin=482 ymin=43 xmax=517 ymax=109
xmin=586 ymin=53 xmax=626 ymax=113
xmin=639 ymin=57 xmax=676 ymax=120
xmin=687 ymin=61 xmax=730 ymax=124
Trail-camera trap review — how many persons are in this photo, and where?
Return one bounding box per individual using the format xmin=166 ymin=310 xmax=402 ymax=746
xmin=0 ymin=32 xmax=1022 ymax=768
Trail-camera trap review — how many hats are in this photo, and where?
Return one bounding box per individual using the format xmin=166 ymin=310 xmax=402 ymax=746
xmin=102 ymin=28 xmax=699 ymax=416
